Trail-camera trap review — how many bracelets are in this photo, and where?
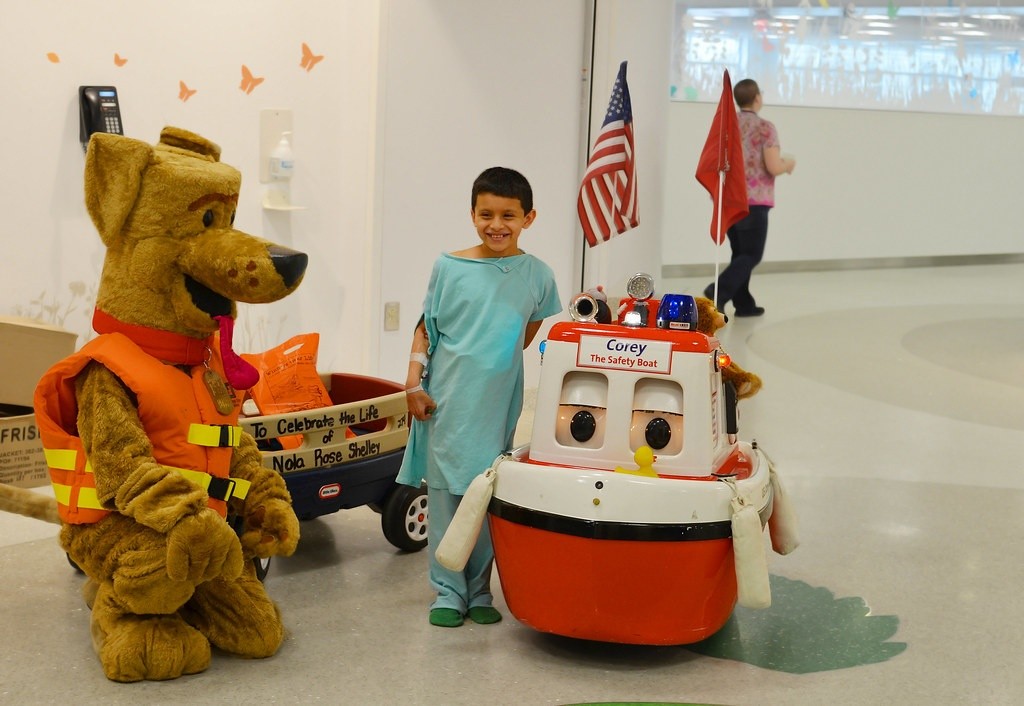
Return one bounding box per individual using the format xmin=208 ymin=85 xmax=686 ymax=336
xmin=409 ymin=352 xmax=429 ymax=369
xmin=405 ymin=383 xmax=425 ymax=395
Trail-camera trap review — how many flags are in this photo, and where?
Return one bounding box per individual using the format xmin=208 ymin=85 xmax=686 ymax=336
xmin=577 ymin=60 xmax=640 ymax=248
xmin=696 ymin=68 xmax=751 ymax=245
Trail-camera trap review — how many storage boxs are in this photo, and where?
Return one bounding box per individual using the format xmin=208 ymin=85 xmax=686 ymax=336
xmin=0 ymin=414 xmax=53 ymax=489
xmin=0 ymin=315 xmax=79 ymax=411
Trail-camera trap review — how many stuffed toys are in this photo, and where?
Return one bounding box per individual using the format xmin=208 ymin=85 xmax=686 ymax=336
xmin=693 ymin=298 xmax=763 ymax=399
xmin=35 ymin=126 xmax=307 ymax=683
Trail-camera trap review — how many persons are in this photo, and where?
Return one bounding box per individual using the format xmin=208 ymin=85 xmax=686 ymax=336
xmin=702 ymin=79 xmax=795 ymax=323
xmin=394 ymin=166 xmax=562 ymax=628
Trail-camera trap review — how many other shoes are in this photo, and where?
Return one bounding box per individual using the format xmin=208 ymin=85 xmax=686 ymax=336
xmin=734 ymin=301 xmax=770 ymax=319
xmin=704 ymin=282 xmax=725 ymax=314
